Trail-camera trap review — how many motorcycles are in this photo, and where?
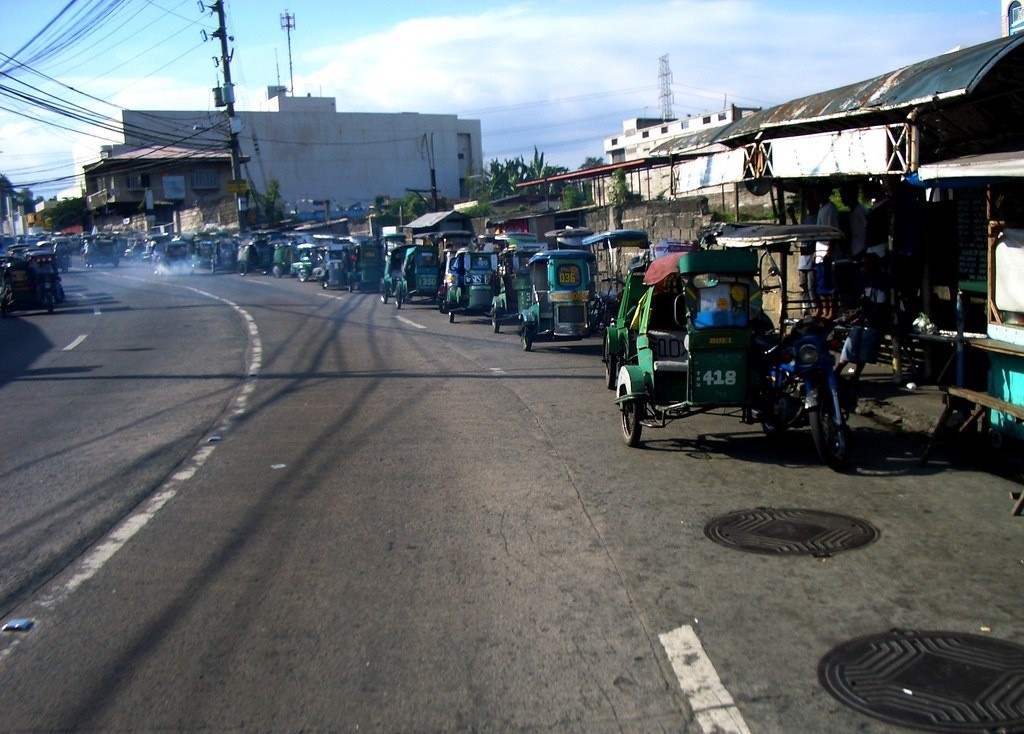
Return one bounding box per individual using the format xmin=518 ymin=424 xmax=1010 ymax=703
xmin=1 ymin=218 xmax=881 ymax=475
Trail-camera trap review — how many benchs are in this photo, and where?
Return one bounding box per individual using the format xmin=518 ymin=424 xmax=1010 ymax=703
xmin=919 ymin=384 xmax=1024 ymax=516
